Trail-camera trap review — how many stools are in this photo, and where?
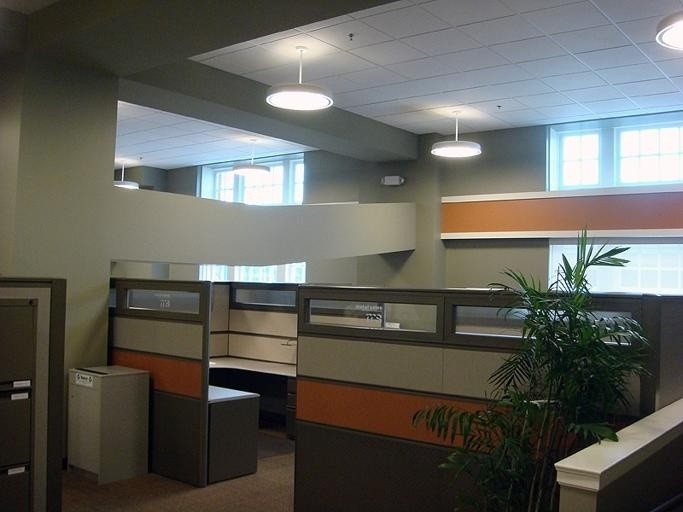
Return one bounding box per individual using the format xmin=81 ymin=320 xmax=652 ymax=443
xmin=205 ymin=381 xmax=260 ymax=486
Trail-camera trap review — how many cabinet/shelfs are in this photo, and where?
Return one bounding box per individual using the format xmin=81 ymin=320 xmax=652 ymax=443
xmin=63 ymin=364 xmax=151 ymax=486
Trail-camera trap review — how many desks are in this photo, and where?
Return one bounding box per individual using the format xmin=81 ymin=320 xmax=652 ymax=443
xmin=202 ymin=356 xmax=296 ymax=441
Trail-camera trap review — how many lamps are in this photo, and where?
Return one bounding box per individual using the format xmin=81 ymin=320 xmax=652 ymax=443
xmin=650 ymin=9 xmax=683 ymax=51
xmin=230 ymin=139 xmax=273 ymax=177
xmin=256 ymin=45 xmax=336 ymax=114
xmin=428 ymin=108 xmax=484 ymax=161
xmin=113 ymin=157 xmax=142 ymax=191
